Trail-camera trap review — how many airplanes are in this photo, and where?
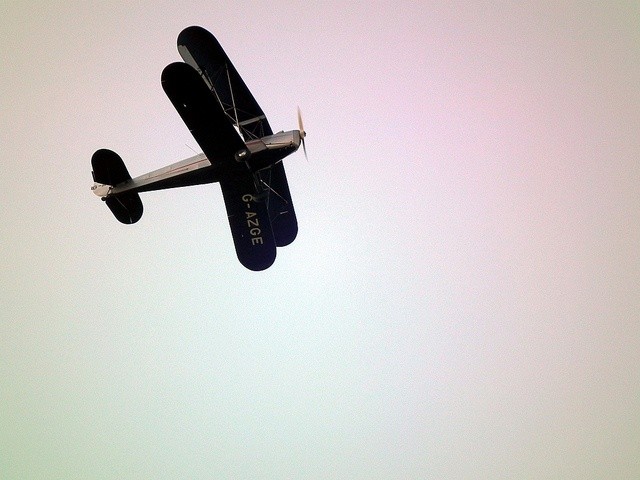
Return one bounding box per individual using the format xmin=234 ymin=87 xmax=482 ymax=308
xmin=90 ymin=23 xmax=310 ymax=272
